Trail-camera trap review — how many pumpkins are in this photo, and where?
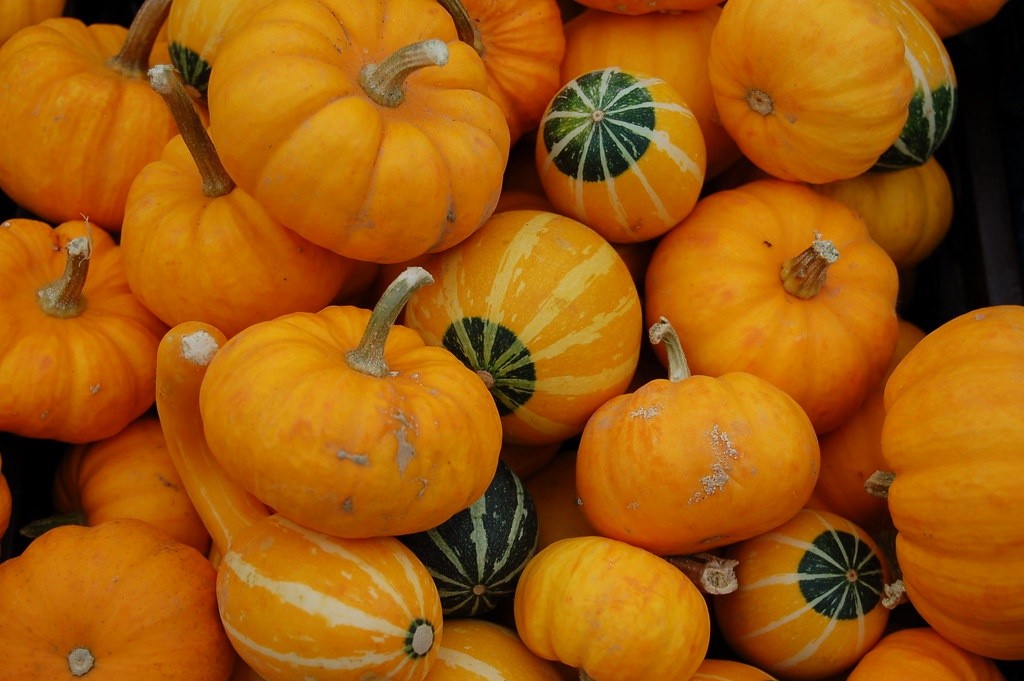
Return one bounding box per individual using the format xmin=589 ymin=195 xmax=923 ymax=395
xmin=0 ymin=0 xmax=1024 ymax=681
